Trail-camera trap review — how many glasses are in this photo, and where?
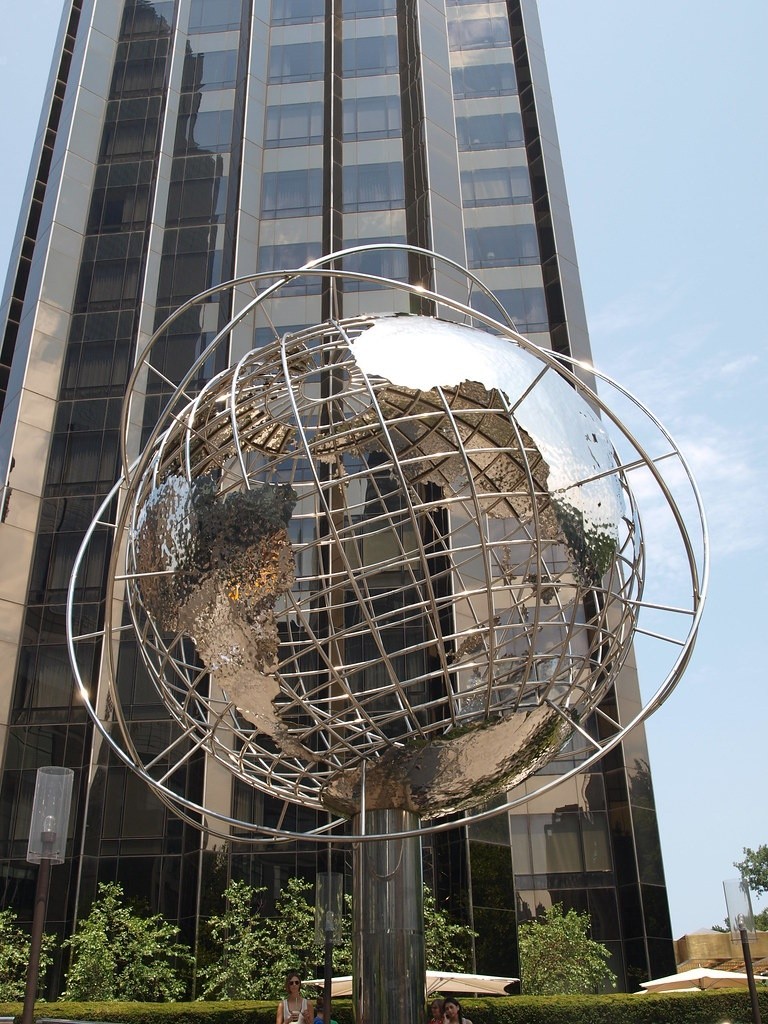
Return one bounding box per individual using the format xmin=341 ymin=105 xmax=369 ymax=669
xmin=290 ymin=981 xmax=300 ymax=985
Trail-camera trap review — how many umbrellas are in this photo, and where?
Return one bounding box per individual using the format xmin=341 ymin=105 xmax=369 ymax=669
xmin=301 ymin=970 xmax=520 ymax=1014
xmin=638 ymin=964 xmax=768 ymax=991
xmin=634 ymin=987 xmax=701 ymax=995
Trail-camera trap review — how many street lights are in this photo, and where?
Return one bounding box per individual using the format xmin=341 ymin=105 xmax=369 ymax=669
xmin=314 ymin=873 xmax=344 ymax=1024
xmin=21 ymin=766 xmax=75 ymax=1024
xmin=721 ymin=876 xmax=763 ymax=1024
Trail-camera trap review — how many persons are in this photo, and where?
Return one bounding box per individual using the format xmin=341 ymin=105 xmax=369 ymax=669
xmin=314 ymin=997 xmax=333 ymax=1024
xmin=276 ymin=972 xmax=315 ymax=1024
xmin=443 ymin=997 xmax=472 ymax=1024
xmin=430 ymin=999 xmax=445 ymax=1024
